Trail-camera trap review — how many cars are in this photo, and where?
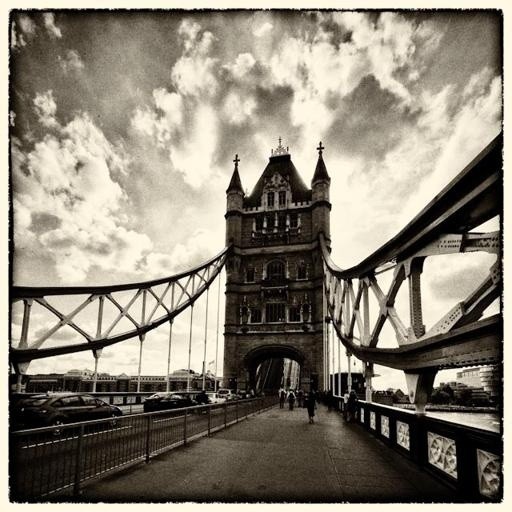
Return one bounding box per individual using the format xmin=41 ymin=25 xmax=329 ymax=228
xmin=205 ymin=392 xmax=227 ymax=404
xmin=143 ymin=392 xmax=196 ymax=419
xmin=19 ymin=391 xmax=124 ymax=437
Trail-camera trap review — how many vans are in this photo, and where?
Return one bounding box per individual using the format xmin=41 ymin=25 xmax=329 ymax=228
xmin=218 ymin=388 xmax=243 ymax=402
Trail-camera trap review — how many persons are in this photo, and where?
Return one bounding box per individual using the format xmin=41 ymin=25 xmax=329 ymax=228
xmin=343 ymin=390 xmax=358 ymax=421
xmin=278 ymin=384 xmax=315 ymax=424
